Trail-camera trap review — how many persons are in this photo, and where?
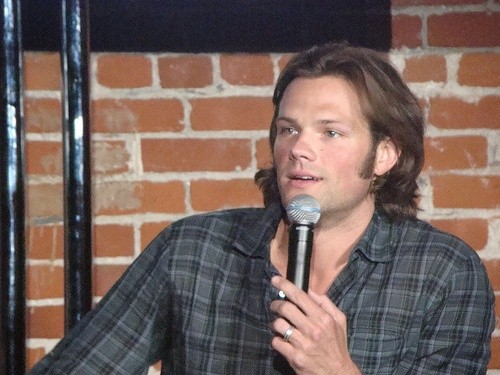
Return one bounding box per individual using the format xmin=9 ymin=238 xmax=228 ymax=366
xmin=24 ymin=40 xmax=496 ymax=375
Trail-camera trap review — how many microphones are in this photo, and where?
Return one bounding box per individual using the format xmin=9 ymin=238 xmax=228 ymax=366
xmin=284 ymin=194 xmax=321 ymax=333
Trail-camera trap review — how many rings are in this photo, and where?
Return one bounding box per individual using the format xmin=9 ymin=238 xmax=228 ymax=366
xmin=283 ymin=325 xmax=294 ymax=342
